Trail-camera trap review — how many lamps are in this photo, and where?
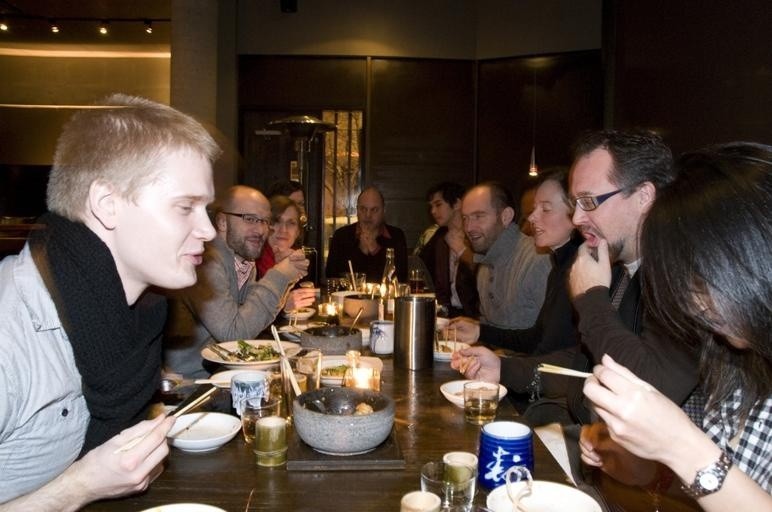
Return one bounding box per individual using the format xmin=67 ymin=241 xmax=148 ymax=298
xmin=524 ymin=55 xmax=543 ymax=179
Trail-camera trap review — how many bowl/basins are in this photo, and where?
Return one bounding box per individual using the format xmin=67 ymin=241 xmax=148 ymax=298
xmin=301 ymin=327 xmax=362 ymax=355
xmin=436 ymin=317 xmax=450 ymax=331
xmin=344 ymin=294 xmax=380 ymax=317
xmin=440 ymin=380 xmax=508 ymax=408
xmin=378 ymin=248 xmax=400 ymax=321
xmin=357 ymin=328 xmax=371 ymax=348
xmin=293 ymin=386 xmax=396 ymax=456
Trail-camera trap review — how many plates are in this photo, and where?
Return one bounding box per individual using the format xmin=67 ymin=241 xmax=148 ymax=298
xmin=280 ymin=326 xmax=310 ymax=342
xmin=433 ymin=340 xmax=471 ymax=362
xmin=321 ymin=356 xmax=383 ymax=386
xmin=486 ymin=481 xmax=602 ymax=512
xmin=165 ymin=412 xmax=241 ymax=453
xmin=209 ymin=370 xmax=268 ymax=388
xmin=141 ymin=502 xmax=231 ymax=512
xmin=281 ymin=308 xmax=316 ymax=320
xmin=200 ymin=340 xmax=303 ymax=370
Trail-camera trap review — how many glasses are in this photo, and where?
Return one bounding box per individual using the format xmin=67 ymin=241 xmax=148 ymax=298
xmin=568 ymin=179 xmax=645 ymax=212
xmin=222 ymin=211 xmax=271 ymax=225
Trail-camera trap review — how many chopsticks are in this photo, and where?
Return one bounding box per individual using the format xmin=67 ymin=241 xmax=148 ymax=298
xmin=116 ymin=387 xmax=217 ymax=451
xmin=460 ymin=344 xmax=473 ymax=375
xmin=194 ymin=379 xmax=232 ymax=385
xmin=445 ymin=319 xmax=457 ymax=353
xmin=537 ymin=364 xmax=593 ymax=379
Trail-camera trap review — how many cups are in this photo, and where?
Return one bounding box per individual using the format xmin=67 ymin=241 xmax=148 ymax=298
xmin=394 ymin=296 xmax=436 ymax=370
xmin=478 ymin=421 xmax=534 ymax=491
xmin=369 ymin=321 xmax=395 ymax=354
xmin=399 ymin=451 xmax=478 ymax=512
xmin=345 ymin=272 xmax=366 ymax=291
xmin=231 ymin=348 xmax=322 ymax=468
xmin=317 ymin=277 xmax=411 ymax=319
xmin=301 ymin=248 xmax=319 ymax=282
xmin=345 ymin=367 xmax=380 ymax=392
xmin=464 ymin=381 xmax=499 ymax=427
xmin=410 ymin=270 xmax=424 ymax=294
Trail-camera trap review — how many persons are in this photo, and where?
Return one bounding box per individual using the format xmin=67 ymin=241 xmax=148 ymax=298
xmin=267 ymin=181 xmax=306 ymax=219
xmin=256 ymin=195 xmax=320 ymax=318
xmin=324 ymin=186 xmax=409 ymax=292
xmin=0 ymin=92 xmax=217 ymax=511
xmin=163 ymin=185 xmax=310 ymax=378
